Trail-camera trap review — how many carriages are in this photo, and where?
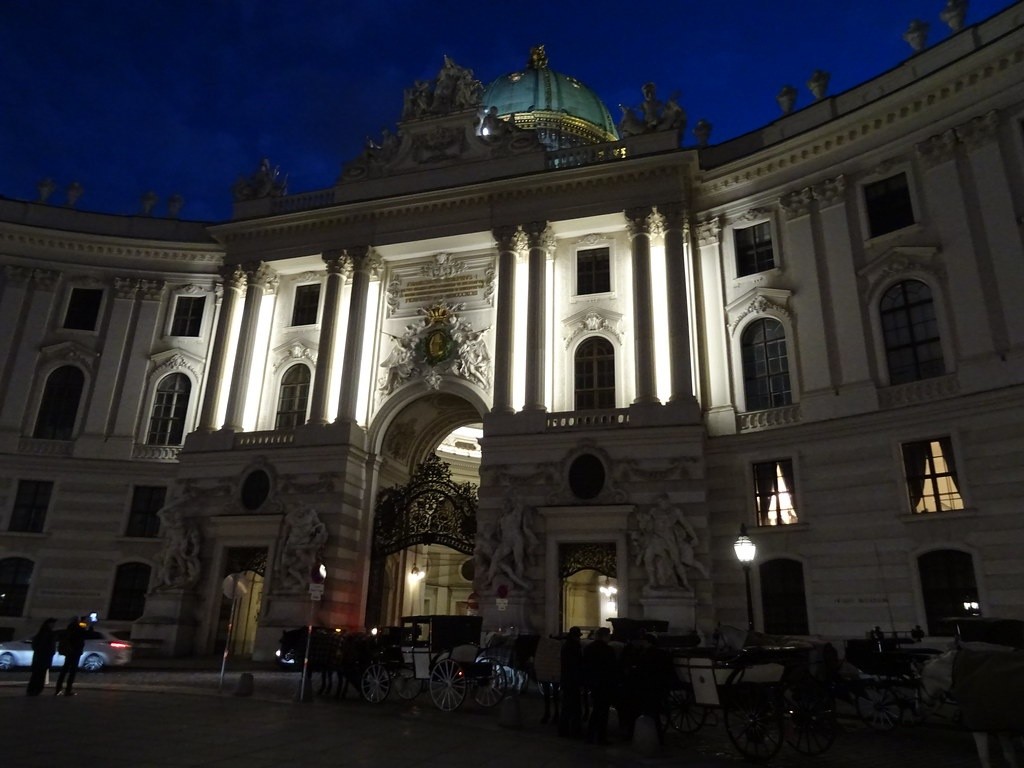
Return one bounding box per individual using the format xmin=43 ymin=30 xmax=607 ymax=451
xmin=707 ymin=618 xmax=948 ymax=733
xmin=278 ymin=615 xmax=508 ymax=713
xmin=488 ymin=615 xmax=840 ymax=762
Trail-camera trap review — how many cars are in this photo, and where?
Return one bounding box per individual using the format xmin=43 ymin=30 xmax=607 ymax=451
xmin=0 ymin=622 xmax=134 ymax=671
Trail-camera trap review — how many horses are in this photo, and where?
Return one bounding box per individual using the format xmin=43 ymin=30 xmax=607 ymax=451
xmin=276 ymin=626 xmax=380 ymax=704
xmin=484 ymin=626 xmax=626 ymax=738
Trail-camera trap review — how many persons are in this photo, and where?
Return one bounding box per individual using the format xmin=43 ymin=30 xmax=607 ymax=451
xmin=156 ymin=497 xmax=202 ymax=587
xmin=254 ymin=163 xmax=273 ymax=191
xmin=632 ymin=491 xmax=701 ymax=595
xmin=381 ymin=129 xmax=400 ymax=147
xmin=278 ymin=500 xmax=327 ymax=595
xmin=482 ymin=494 xmax=545 ymax=588
xmin=560 ymin=625 xmax=619 ymax=747
xmin=629 ymin=82 xmax=670 ymax=129
xmin=411 ymin=55 xmax=487 ymax=115
xmin=377 ymin=336 xmax=415 ymax=394
xmin=24 ymin=614 xmax=94 ymax=698
xmin=456 ymin=328 xmax=488 ymax=385
xmin=481 ymin=106 xmax=523 ymax=136
